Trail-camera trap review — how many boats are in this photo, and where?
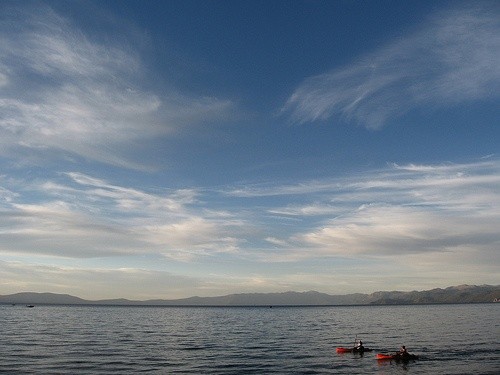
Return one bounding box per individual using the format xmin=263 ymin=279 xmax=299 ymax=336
xmin=376 ymin=353 xmax=418 ymax=360
xmin=336 ymin=348 xmax=372 ymax=353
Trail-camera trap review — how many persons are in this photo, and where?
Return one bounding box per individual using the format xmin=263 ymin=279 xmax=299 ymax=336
xmin=357 ymin=340 xmax=364 ymax=349
xmin=399 ymin=346 xmax=407 ymax=356
